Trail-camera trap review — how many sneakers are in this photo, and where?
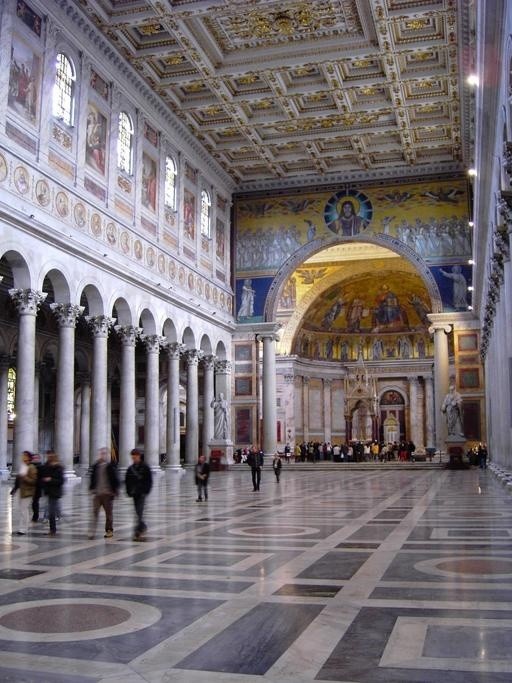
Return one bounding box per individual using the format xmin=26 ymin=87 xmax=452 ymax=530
xmin=13 ymin=512 xmax=64 ymax=538
xmin=104 ymin=528 xmax=113 ymax=538
xmin=133 ymin=522 xmax=148 ymax=539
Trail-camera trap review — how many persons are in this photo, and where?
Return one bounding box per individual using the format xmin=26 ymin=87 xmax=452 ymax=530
xmin=233 ymin=446 xmax=264 ymax=464
xmin=437 ymin=264 xmax=473 ymax=312
xmin=124 ymin=447 xmax=153 ymax=542
xmin=194 ymin=455 xmax=211 ymax=502
xmin=382 ymin=212 xmax=472 ymax=258
xmin=272 ymin=452 xmax=282 ymax=483
xmin=247 ymin=445 xmax=264 ymax=491
xmin=85 ymin=445 xmax=122 ymax=540
xmin=235 ymin=279 xmax=256 ymax=322
xmin=324 ymin=199 xmax=366 ymax=237
xmin=294 ymin=439 xmax=415 ymax=464
xmin=441 ymin=384 xmax=465 ymax=437
xmin=283 ymin=442 xmax=291 ymax=463
xmin=236 ymin=214 xmax=317 ymax=270
xmin=290 ymin=282 xmax=436 ymax=361
xmin=210 ymin=391 xmax=232 ymax=440
xmin=468 ymin=442 xmax=489 ymax=468
xmin=9 ymin=448 xmax=65 ymax=538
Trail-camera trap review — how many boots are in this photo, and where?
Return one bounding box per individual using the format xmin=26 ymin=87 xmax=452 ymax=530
xmin=204 ymin=494 xmax=208 ymax=501
xmin=196 ymin=495 xmax=202 ymax=502
